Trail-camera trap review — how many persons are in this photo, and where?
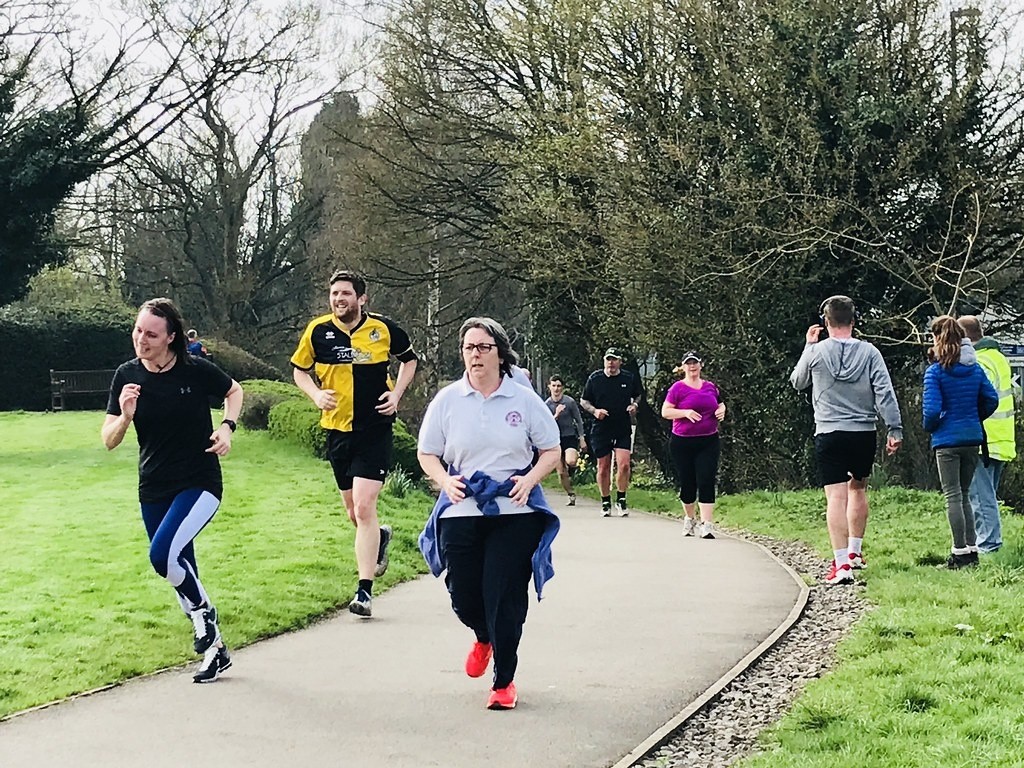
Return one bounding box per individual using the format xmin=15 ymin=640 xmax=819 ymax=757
xmin=662 ymin=352 xmax=726 ymax=538
xmin=545 ymin=375 xmax=587 ymax=505
xmin=187 ymin=330 xmax=206 ymax=356
xmin=581 ymin=348 xmax=643 ymax=514
xmin=510 ymin=363 xmax=535 ymax=392
xmin=290 ymin=273 xmax=418 ymax=615
xmin=101 ymin=298 xmax=244 ymax=682
xmin=791 ymin=297 xmax=902 ymax=584
xmin=417 ymin=318 xmax=561 ymax=709
xmin=923 ymin=315 xmax=998 ymax=570
xmin=956 ymin=316 xmax=1016 ymax=552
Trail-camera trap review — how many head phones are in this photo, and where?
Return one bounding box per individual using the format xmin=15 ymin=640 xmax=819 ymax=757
xmin=819 ymin=295 xmax=859 ymax=326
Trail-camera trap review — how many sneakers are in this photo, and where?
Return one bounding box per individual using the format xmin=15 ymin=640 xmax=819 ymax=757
xmin=193 ymin=642 xmax=233 ymax=684
xmin=465 ymin=640 xmax=493 ymax=678
xmin=847 ymin=553 xmax=868 ymax=569
xmin=699 ymin=522 xmax=715 ymax=539
xmin=601 ymin=502 xmax=612 ymax=517
xmin=567 ymin=492 xmax=575 ymax=506
xmin=683 ymin=516 xmax=696 ymax=536
xmin=373 ymin=525 xmax=393 ymax=577
xmin=566 ymin=465 xmax=578 ymax=477
xmin=487 ymin=681 xmax=518 ymax=709
xmin=936 ymin=545 xmax=972 ymax=569
xmin=348 ymin=589 xmax=373 ymax=616
xmin=968 ymin=544 xmax=980 ymax=567
xmin=191 ymin=606 xmax=218 ymax=655
xmin=614 ymin=498 xmax=629 ymax=517
xmin=825 ymin=560 xmax=854 ymax=585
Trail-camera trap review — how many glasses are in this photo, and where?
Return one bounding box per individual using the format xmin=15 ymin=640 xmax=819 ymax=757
xmin=461 ymin=343 xmax=499 ymax=354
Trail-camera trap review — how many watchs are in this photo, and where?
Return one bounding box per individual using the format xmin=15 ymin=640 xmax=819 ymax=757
xmin=222 ymin=419 xmax=236 ymax=432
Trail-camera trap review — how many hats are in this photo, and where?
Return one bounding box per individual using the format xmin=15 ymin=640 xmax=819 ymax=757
xmin=604 ymin=347 xmax=622 ymax=360
xmin=681 ymin=350 xmax=702 ymax=363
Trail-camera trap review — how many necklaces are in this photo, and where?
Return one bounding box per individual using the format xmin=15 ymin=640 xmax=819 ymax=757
xmin=157 ymin=353 xmax=175 ymax=372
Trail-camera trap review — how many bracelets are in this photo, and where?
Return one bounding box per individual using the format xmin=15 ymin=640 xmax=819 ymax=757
xmin=633 ymin=402 xmax=638 ymax=407
